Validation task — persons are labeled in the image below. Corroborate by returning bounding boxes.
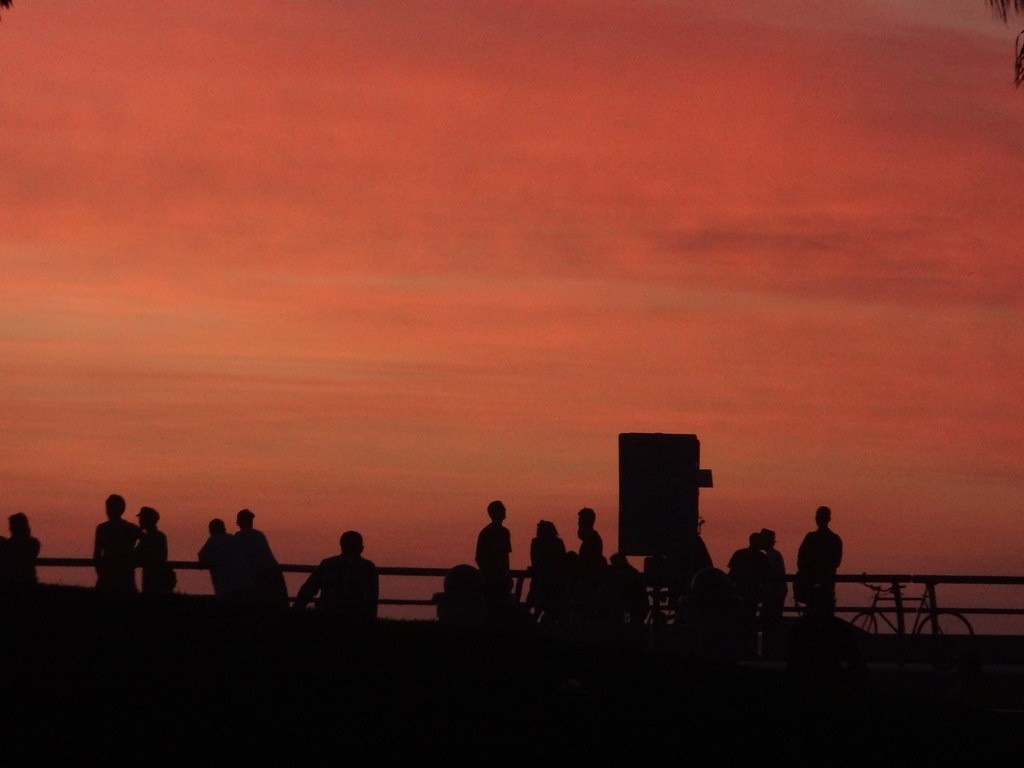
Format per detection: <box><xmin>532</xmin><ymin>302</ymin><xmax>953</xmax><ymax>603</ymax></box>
<box><xmin>523</xmin><ymin>520</ymin><xmax>565</xmax><ymax>622</ymax></box>
<box><xmin>92</xmin><ymin>494</ymin><xmax>139</xmax><ymax>591</ymax></box>
<box><xmin>3</xmin><ymin>513</ymin><xmax>41</xmax><ymax>587</ymax></box>
<box><xmin>131</xmin><ymin>506</ymin><xmax>179</xmax><ymax>594</ymax></box>
<box><xmin>793</xmin><ymin>504</ymin><xmax>842</xmax><ymax>618</ymax></box>
<box><xmin>233</xmin><ymin>509</ymin><xmax>288</xmax><ymax>602</ymax></box>
<box><xmin>756</xmin><ymin>528</ymin><xmax>788</xmax><ymax>617</ymax></box>
<box><xmin>475</xmin><ymin>501</ymin><xmax>514</xmax><ymax>620</ymax></box>
<box><xmin>567</xmin><ymin>507</ymin><xmax>653</xmax><ymax>628</ymax></box>
<box><xmin>725</xmin><ymin>532</ymin><xmax>766</xmax><ymax>622</ymax></box>
<box><xmin>198</xmin><ymin>515</ymin><xmax>250</xmax><ymax>593</ymax></box>
<box><xmin>292</xmin><ymin>526</ymin><xmax>380</xmax><ymax>618</ymax></box>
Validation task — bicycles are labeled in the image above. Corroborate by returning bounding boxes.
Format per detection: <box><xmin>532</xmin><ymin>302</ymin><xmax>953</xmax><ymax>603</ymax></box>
<box><xmin>848</xmin><ymin>572</ymin><xmax>978</xmax><ymax>663</ymax></box>
